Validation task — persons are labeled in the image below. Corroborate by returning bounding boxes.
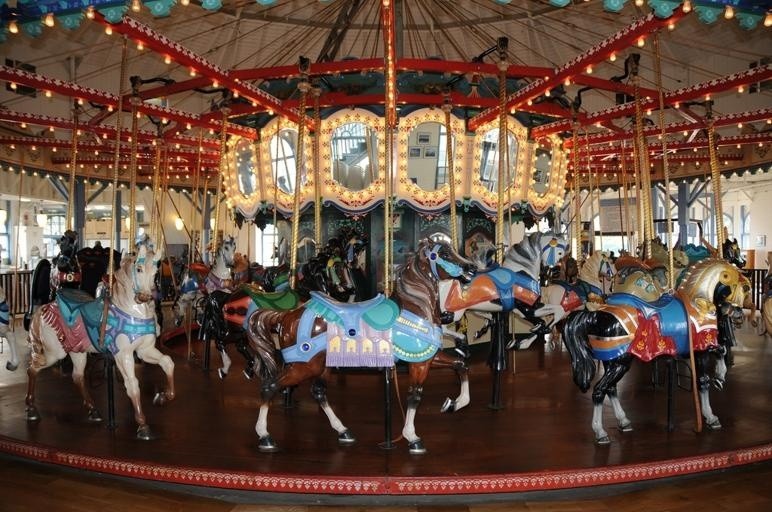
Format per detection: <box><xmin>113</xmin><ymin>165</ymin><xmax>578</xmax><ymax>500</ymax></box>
<box><xmin>278</xmin><ymin>176</ymin><xmax>287</xmax><ymax>190</ymax></box>
